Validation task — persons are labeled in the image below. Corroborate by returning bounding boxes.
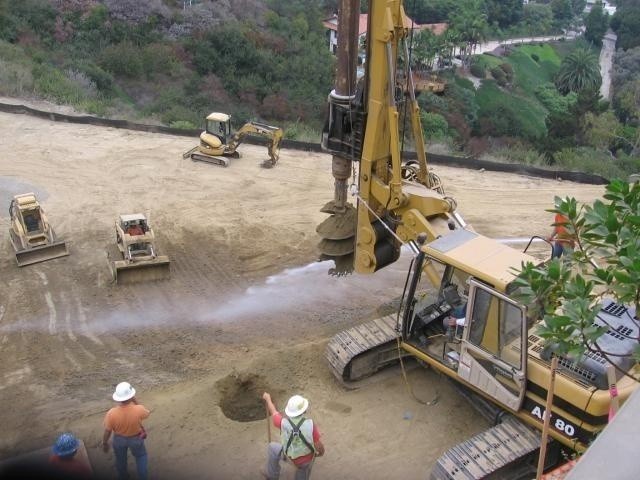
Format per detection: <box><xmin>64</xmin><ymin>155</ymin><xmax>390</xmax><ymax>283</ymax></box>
<box><xmin>102</xmin><ymin>381</ymin><xmax>151</xmax><ymax>480</ymax></box>
<box><xmin>441</xmin><ymin>274</ymin><xmax>488</xmax><ymax>336</ymax></box>
<box><xmin>218</xmin><ymin>122</ymin><xmax>225</xmax><ymax>133</ymax></box>
<box><xmin>546</xmin><ymin>204</ymin><xmax>580</xmax><ymax>267</ymax></box>
<box><xmin>47</xmin><ymin>430</ymin><xmax>93</xmax><ymax>480</ymax></box>
<box><xmin>125</xmin><ymin>222</ymin><xmax>146</xmax><ymax>251</ymax></box>
<box><xmin>260</xmin><ymin>390</ymin><xmax>325</xmax><ymax>480</ymax></box>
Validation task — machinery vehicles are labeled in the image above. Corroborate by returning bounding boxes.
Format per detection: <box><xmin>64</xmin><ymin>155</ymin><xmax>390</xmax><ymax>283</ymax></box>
<box><xmin>183</xmin><ymin>112</ymin><xmax>283</xmax><ymax>169</ymax></box>
<box><xmin>314</xmin><ymin>1</ymin><xmax>640</xmax><ymax>463</ymax></box>
<box><xmin>112</xmin><ymin>213</ymin><xmax>171</xmax><ymax>285</ymax></box>
<box><xmin>7</xmin><ymin>192</ymin><xmax>70</xmax><ymax>268</ymax></box>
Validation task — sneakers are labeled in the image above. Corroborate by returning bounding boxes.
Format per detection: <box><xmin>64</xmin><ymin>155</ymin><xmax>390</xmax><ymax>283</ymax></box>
<box><xmin>260</xmin><ymin>465</ymin><xmax>277</xmax><ymax>478</ymax></box>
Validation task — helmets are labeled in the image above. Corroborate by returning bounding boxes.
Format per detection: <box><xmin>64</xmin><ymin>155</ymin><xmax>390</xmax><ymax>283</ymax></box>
<box><xmin>285</xmin><ymin>395</ymin><xmax>308</xmax><ymax>418</ymax></box>
<box><xmin>52</xmin><ymin>433</ymin><xmax>80</xmax><ymax>457</ymax></box>
<box><xmin>112</xmin><ymin>381</ymin><xmax>136</xmax><ymax>402</ymax></box>
